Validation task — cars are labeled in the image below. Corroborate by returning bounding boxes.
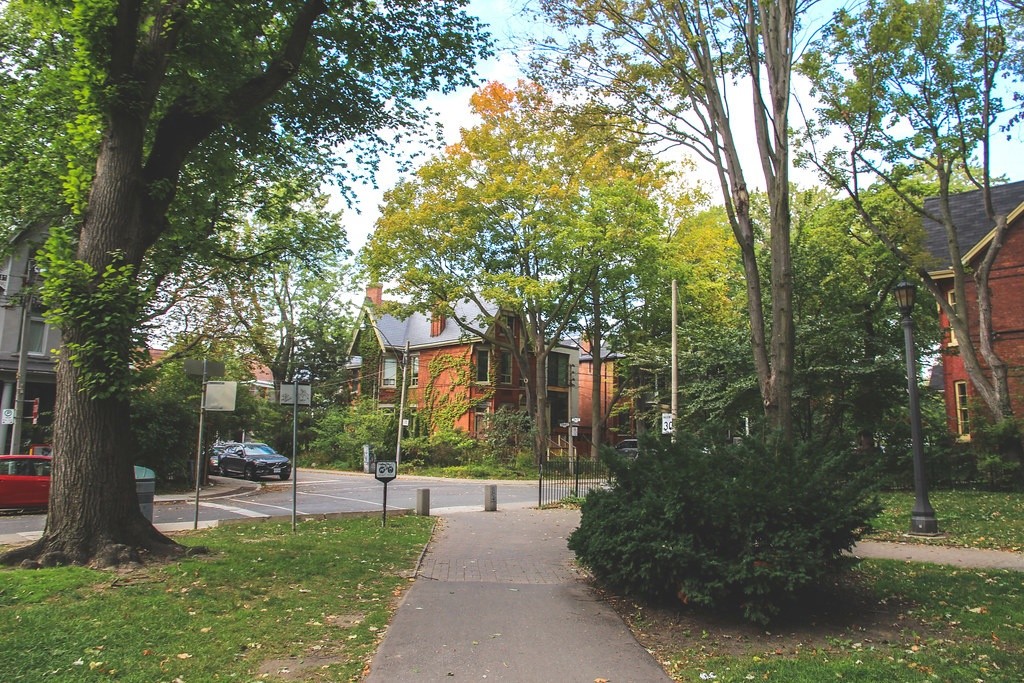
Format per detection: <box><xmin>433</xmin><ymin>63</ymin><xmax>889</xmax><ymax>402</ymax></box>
<box><xmin>614</xmin><ymin>438</ymin><xmax>657</xmax><ymax>460</ymax></box>
<box><xmin>0</xmin><ymin>454</ymin><xmax>52</xmax><ymax>506</ymax></box>
<box><xmin>208</xmin><ymin>443</ymin><xmax>227</xmax><ymax>475</ymax></box>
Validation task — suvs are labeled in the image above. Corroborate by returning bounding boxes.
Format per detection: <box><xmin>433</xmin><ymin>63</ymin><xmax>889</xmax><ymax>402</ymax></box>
<box><xmin>216</xmin><ymin>443</ymin><xmax>292</xmax><ymax>482</ymax></box>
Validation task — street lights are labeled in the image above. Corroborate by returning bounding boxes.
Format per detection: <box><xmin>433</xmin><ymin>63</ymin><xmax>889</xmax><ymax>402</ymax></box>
<box><xmin>890</xmin><ymin>279</ymin><xmax>943</xmax><ymax>537</ymax></box>
<box><xmin>358</xmin><ymin>320</ymin><xmax>411</xmax><ymax>472</ymax></box>
<box><xmin>192</xmin><ymin>338</ymin><xmax>213</xmax><ymax>530</ymax></box>
<box><xmin>6</xmin><ymin>260</ymin><xmax>50</xmax><ymax>473</ymax></box>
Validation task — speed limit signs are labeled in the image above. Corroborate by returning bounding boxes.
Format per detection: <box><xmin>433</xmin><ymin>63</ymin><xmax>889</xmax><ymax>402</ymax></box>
<box><xmin>661</xmin><ymin>413</ymin><xmax>673</xmax><ymax>435</ymax></box>
